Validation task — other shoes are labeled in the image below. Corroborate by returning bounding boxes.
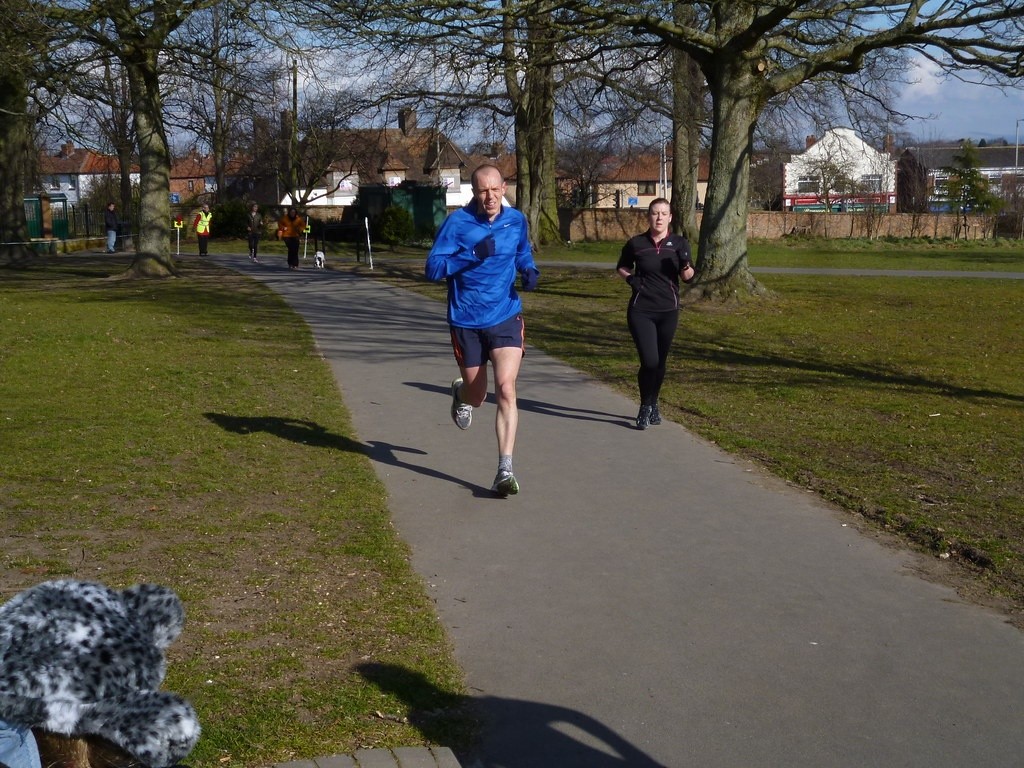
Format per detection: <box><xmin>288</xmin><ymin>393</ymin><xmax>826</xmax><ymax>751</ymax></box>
<box><xmin>253</xmin><ymin>257</ymin><xmax>258</xmax><ymax>263</ymax></box>
<box><xmin>289</xmin><ymin>265</ymin><xmax>297</xmax><ymax>270</ymax></box>
<box><xmin>107</xmin><ymin>249</ymin><xmax>118</xmax><ymax>254</ymax></box>
<box><xmin>249</xmin><ymin>252</ymin><xmax>252</xmax><ymax>260</ymax></box>
<box><xmin>200</xmin><ymin>252</ymin><xmax>209</xmax><ymax>256</ymax></box>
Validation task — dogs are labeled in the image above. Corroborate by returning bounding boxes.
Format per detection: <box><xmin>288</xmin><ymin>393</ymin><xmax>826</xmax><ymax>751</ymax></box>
<box><xmin>314</xmin><ymin>251</ymin><xmax>325</xmax><ymax>268</ymax></box>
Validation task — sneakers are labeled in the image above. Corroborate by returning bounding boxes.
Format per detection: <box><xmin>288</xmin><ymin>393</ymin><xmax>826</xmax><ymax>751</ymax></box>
<box><xmin>650</xmin><ymin>403</ymin><xmax>661</xmax><ymax>424</ymax></box>
<box><xmin>636</xmin><ymin>404</ymin><xmax>652</xmax><ymax>430</ymax></box>
<box><xmin>490</xmin><ymin>468</ymin><xmax>520</xmax><ymax>499</ymax></box>
<box><xmin>450</xmin><ymin>376</ymin><xmax>473</xmax><ymax>430</ymax></box>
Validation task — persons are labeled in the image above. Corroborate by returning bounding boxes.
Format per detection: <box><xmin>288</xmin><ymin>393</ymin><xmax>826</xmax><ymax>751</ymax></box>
<box><xmin>193</xmin><ymin>204</ymin><xmax>213</xmax><ymax>256</ymax></box>
<box><xmin>105</xmin><ymin>202</ymin><xmax>118</xmax><ymax>253</ymax></box>
<box><xmin>617</xmin><ymin>198</ymin><xmax>696</xmax><ymax>430</ymax></box>
<box><xmin>278</xmin><ymin>208</ymin><xmax>307</xmax><ymax>269</ymax></box>
<box><xmin>245</xmin><ymin>203</ymin><xmax>265</xmax><ymax>264</ymax></box>
<box><xmin>425</xmin><ymin>165</ymin><xmax>540</xmax><ymax>495</ymax></box>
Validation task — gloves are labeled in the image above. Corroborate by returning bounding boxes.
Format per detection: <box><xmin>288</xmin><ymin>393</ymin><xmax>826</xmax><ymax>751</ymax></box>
<box><xmin>676</xmin><ymin>249</ymin><xmax>689</xmax><ymax>269</ymax></box>
<box><xmin>625</xmin><ymin>275</ymin><xmax>643</xmax><ymax>293</ymax></box>
<box><xmin>520</xmin><ymin>270</ymin><xmax>537</xmax><ymax>291</ymax></box>
<box><xmin>473</xmin><ymin>232</ymin><xmax>496</xmax><ymax>260</ymax></box>
